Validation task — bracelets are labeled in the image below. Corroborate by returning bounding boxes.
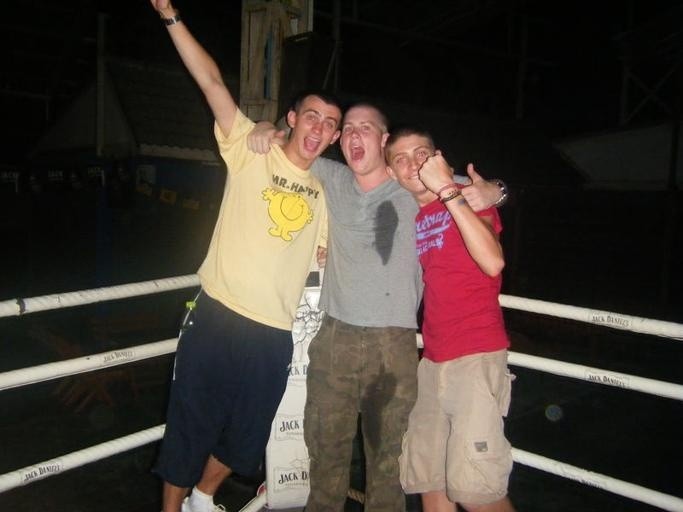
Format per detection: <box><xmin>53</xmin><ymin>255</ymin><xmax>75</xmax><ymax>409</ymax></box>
<box><xmin>161</xmin><ymin>8</ymin><xmax>180</xmax><ymax>25</ymax></box>
<box><xmin>437</xmin><ymin>184</ymin><xmax>461</xmax><ymax>204</ymax></box>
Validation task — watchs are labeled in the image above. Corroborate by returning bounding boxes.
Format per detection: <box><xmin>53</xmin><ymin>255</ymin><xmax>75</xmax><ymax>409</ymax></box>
<box><xmin>489</xmin><ymin>179</ymin><xmax>507</xmax><ymax>207</ymax></box>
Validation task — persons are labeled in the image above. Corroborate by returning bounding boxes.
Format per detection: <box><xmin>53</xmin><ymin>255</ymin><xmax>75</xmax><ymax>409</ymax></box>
<box><xmin>151</xmin><ymin>0</ymin><xmax>326</xmax><ymax>512</ymax></box>
<box><xmin>244</xmin><ymin>101</ymin><xmax>510</xmax><ymax>512</ymax></box>
<box><xmin>317</xmin><ymin>129</ymin><xmax>517</xmax><ymax>512</ymax></box>
